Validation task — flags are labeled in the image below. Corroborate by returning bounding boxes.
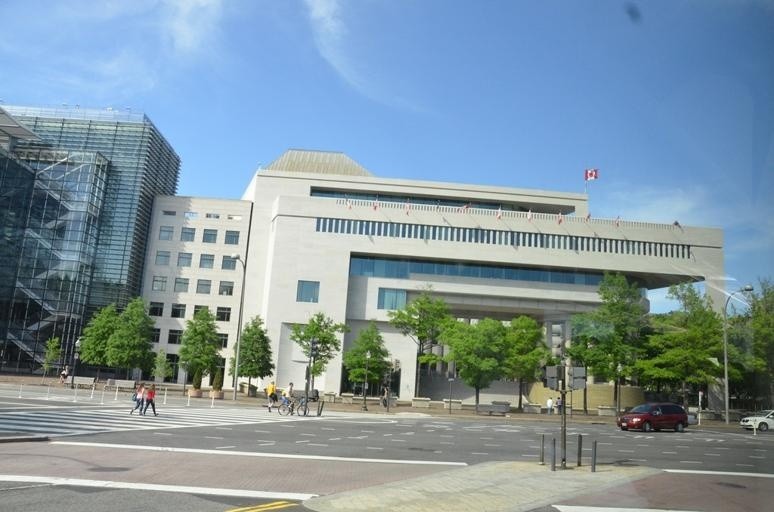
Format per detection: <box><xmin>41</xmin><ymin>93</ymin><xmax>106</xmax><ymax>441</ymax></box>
<box><xmin>585</xmin><ymin>169</ymin><xmax>598</xmax><ymax>181</ymax></box>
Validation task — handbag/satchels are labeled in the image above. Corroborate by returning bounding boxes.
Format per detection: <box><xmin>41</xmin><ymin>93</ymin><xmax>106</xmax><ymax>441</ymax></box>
<box><xmin>269</xmin><ymin>393</ymin><xmax>278</xmax><ymax>403</ymax></box>
<box><xmin>132</xmin><ymin>393</ymin><xmax>136</xmax><ymax>401</ymax></box>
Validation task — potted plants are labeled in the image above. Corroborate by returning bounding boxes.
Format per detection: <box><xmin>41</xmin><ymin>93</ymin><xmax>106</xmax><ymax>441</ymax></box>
<box><xmin>209</xmin><ymin>366</ymin><xmax>224</xmax><ymax>399</ymax></box>
<box><xmin>188</xmin><ymin>367</ymin><xmax>203</xmax><ymax>398</ymax></box>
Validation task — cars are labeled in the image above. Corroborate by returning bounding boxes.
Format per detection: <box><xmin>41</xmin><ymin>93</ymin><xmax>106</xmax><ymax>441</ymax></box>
<box><xmin>739</xmin><ymin>409</ymin><xmax>774</xmax><ymax>432</ymax></box>
<box><xmin>615</xmin><ymin>402</ymin><xmax>698</xmax><ymax>432</ymax></box>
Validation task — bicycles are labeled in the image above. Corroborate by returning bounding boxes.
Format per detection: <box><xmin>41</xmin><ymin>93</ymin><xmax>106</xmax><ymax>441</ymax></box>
<box><xmin>277</xmin><ymin>395</ymin><xmax>309</xmax><ymax>417</ymax></box>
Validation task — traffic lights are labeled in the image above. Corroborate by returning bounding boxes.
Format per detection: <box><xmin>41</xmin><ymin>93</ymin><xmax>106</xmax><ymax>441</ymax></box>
<box><xmin>545</xmin><ymin>320</ymin><xmax>586</xmax><ymax>392</ymax></box>
<box><xmin>309</xmin><ymin>338</ymin><xmax>320</xmax><ymax>357</ymax></box>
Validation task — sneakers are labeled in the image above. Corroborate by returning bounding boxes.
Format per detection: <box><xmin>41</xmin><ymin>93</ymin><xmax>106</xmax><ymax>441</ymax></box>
<box><xmin>130</xmin><ymin>412</ymin><xmax>159</xmax><ymax>416</ymax></box>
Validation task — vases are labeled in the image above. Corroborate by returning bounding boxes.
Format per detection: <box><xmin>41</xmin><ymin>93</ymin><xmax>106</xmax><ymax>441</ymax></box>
<box><xmin>696</xmin><ymin>409</ymin><xmax>742</xmax><ymax>421</ymax></box>
<box><xmin>239</xmin><ymin>381</ymin><xmax>618</xmax><ymax>418</ymax></box>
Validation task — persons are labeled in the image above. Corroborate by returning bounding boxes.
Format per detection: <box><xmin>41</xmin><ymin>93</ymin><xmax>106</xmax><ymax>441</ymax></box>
<box><xmin>143</xmin><ymin>384</ymin><xmax>159</xmax><ymax>416</ymax></box>
<box><xmin>267</xmin><ymin>380</ymin><xmax>276</xmax><ymax>412</ymax></box>
<box><xmin>381</xmin><ymin>384</ymin><xmax>389</xmax><ymax>407</ymax></box>
<box><xmin>383</xmin><ymin>385</ymin><xmax>388</xmax><ymax>409</ymax></box>
<box><xmin>546</xmin><ymin>397</ymin><xmax>554</xmax><ymax>414</ymax></box>
<box><xmin>285</xmin><ymin>382</ymin><xmax>295</xmax><ymax>415</ymax></box>
<box><xmin>556</xmin><ymin>397</ymin><xmax>562</xmax><ymax>415</ymax></box>
<box><xmin>130</xmin><ymin>382</ymin><xmax>145</xmax><ymax>416</ymax></box>
<box><xmin>61</xmin><ymin>369</ymin><xmax>67</xmax><ymax>384</ymax></box>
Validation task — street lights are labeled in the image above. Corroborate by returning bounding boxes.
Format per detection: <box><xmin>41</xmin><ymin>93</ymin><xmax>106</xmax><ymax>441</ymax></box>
<box><xmin>361</xmin><ymin>350</ymin><xmax>372</xmax><ymax>412</ymax></box>
<box><xmin>723</xmin><ymin>285</ymin><xmax>754</xmax><ymax>424</ymax></box>
<box><xmin>70</xmin><ymin>338</ymin><xmax>81</xmax><ymax>388</ymax></box>
<box><xmin>232</xmin><ymin>253</ymin><xmax>246</xmax><ymax>401</ymax></box>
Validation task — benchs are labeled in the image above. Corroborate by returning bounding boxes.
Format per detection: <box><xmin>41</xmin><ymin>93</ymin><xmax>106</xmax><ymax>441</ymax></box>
<box><xmin>64</xmin><ymin>375</ymin><xmax>96</xmax><ymax>390</ymax></box>
<box><xmin>104</xmin><ymin>378</ymin><xmax>136</xmax><ymax>393</ymax></box>
